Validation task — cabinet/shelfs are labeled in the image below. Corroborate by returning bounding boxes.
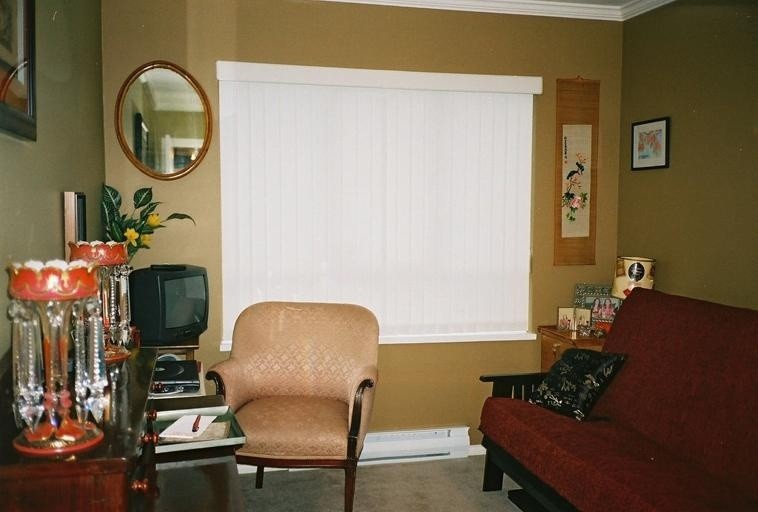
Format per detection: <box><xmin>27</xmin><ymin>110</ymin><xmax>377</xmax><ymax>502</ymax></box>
<box><xmin>536</xmin><ymin>326</ymin><xmax>608</xmax><ymax>372</ymax></box>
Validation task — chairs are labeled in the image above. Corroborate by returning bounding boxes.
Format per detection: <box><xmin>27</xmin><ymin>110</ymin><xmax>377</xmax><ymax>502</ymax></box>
<box><xmin>205</xmin><ymin>296</ymin><xmax>381</xmax><ymax>512</ymax></box>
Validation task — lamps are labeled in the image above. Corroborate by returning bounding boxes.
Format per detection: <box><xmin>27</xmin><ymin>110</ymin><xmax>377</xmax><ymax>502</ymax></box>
<box><xmin>611</xmin><ymin>255</ymin><xmax>656</xmax><ymax>302</ymax></box>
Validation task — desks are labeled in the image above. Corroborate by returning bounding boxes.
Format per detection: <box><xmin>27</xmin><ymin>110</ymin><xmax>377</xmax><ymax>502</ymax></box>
<box><xmin>0</xmin><ymin>330</ymin><xmax>244</xmax><ymax>512</ymax></box>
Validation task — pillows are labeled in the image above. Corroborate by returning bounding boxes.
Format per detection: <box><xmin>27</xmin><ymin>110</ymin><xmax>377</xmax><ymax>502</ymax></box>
<box><xmin>532</xmin><ymin>345</ymin><xmax>629</xmax><ymax>416</ymax></box>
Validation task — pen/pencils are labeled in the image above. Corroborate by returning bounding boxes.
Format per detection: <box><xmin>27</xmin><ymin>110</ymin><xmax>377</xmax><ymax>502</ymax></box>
<box><xmin>193</xmin><ymin>415</ymin><xmax>201</xmax><ymax>432</ymax></box>
<box><xmin>151</xmin><ymin>265</ymin><xmax>186</xmax><ymax>271</ymax></box>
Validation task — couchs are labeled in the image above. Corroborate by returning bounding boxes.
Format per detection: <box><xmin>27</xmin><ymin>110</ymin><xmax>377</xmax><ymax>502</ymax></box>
<box><xmin>477</xmin><ymin>286</ymin><xmax>757</xmax><ymax>512</ymax></box>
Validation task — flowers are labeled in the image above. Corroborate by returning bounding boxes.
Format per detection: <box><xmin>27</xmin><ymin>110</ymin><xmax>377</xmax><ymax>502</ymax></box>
<box><xmin>100</xmin><ymin>183</ymin><xmax>197</xmax><ymax>265</ymax></box>
<box><xmin>562</xmin><ymin>152</ymin><xmax>588</xmax><ymax>222</ymax></box>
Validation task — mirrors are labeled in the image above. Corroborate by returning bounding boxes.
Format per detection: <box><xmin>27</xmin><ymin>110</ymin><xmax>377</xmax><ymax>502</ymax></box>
<box><xmin>114</xmin><ymin>59</ymin><xmax>213</xmax><ymax>181</ymax></box>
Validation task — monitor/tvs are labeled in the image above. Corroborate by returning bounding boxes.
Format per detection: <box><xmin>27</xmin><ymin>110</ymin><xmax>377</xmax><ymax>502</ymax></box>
<box><xmin>127</xmin><ymin>265</ymin><xmax>208</xmax><ymax>346</ymax></box>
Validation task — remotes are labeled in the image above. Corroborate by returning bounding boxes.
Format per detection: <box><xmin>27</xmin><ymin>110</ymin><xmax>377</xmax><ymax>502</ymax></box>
<box><xmin>151</xmin><ymin>263</ymin><xmax>186</xmax><ymax>271</ymax></box>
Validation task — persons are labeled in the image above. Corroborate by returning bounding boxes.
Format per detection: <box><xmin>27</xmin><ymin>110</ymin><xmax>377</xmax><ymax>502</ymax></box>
<box><xmin>587</xmin><ymin>297</ymin><xmax>602</xmax><ymax>319</ymax></box>
<box><xmin>575</xmin><ymin>315</ymin><xmax>587</xmax><ymax>327</ymax></box>
<box><xmin>558</xmin><ymin>314</ymin><xmax>569</xmax><ymax>328</ymax></box>
<box><xmin>600</xmin><ymin>298</ymin><xmax>615</xmax><ymax>320</ymax></box>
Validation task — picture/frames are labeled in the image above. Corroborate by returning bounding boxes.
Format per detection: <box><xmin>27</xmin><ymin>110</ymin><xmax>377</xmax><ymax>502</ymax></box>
<box><xmin>135</xmin><ymin>113</ymin><xmax>151</xmax><ymax>171</ymax></box>
<box><xmin>575</xmin><ymin>281</ymin><xmax>620</xmax><ymax>331</ymax></box>
<box><xmin>630</xmin><ymin>116</ymin><xmax>672</xmax><ymax>171</ymax></box>
<box><xmin>0</xmin><ymin>0</ymin><xmax>39</xmax><ymax>140</ymax></box>
